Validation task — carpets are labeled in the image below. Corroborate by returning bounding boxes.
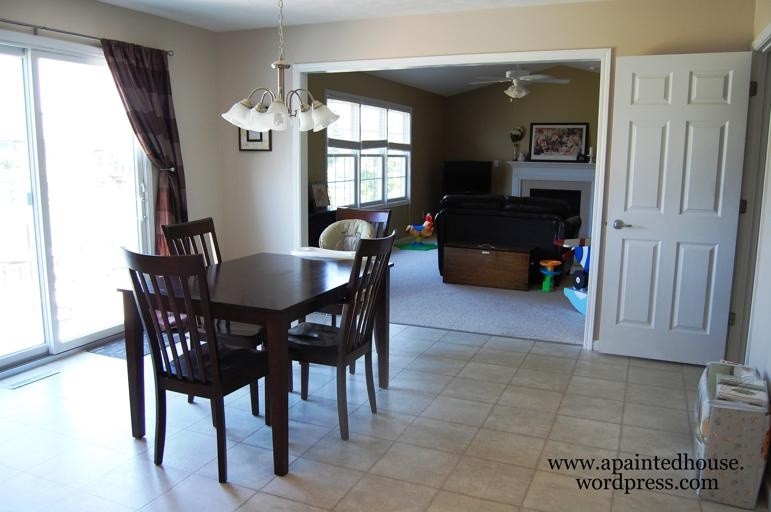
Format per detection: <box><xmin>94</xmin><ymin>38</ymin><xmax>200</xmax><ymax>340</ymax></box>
<box><xmin>386</xmin><ymin>229</ymin><xmax>584</xmax><ymax>348</ymax></box>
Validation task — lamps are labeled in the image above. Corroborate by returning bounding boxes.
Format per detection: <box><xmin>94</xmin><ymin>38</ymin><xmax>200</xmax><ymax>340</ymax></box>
<box><xmin>504</xmin><ymin>81</ymin><xmax>531</xmax><ymax>101</ymax></box>
<box><xmin>218</xmin><ymin>0</ymin><xmax>338</xmax><ymax>135</ymax></box>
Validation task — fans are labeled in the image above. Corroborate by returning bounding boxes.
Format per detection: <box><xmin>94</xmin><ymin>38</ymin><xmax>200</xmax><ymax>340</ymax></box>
<box><xmin>466</xmin><ymin>62</ymin><xmax>571</xmax><ymax>85</ymax></box>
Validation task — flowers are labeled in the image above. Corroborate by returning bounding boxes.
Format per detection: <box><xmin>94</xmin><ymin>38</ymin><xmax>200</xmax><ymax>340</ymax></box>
<box><xmin>506</xmin><ymin>126</ymin><xmax>525</xmax><ymax>141</ymax></box>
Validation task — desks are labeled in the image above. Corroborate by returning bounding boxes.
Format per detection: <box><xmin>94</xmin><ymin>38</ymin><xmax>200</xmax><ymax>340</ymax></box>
<box><xmin>115</xmin><ymin>251</ymin><xmax>395</xmax><ymax>478</ymax></box>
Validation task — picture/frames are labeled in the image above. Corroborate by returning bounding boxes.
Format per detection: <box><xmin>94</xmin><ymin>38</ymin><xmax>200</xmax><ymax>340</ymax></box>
<box><xmin>237</xmin><ymin>105</ymin><xmax>271</xmax><ymax>153</ymax></box>
<box><xmin>528</xmin><ymin>121</ymin><xmax>590</xmax><ymax>163</ymax></box>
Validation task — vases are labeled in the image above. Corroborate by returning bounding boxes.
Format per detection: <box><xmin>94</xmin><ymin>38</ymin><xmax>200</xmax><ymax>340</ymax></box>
<box><xmin>512</xmin><ymin>142</ymin><xmax>518</xmax><ymax>161</ymax></box>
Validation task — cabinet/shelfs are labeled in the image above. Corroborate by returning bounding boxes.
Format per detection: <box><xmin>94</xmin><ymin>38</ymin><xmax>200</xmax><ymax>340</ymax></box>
<box><xmin>119</xmin><ymin>244</ymin><xmax>272</xmax><ymax>485</ymax></box>
<box><xmin>285</xmin><ymin>230</ymin><xmax>405</xmax><ymax>446</ymax></box>
<box><xmin>159</xmin><ymin>216</ymin><xmax>292</xmax><ymax>418</ymax></box>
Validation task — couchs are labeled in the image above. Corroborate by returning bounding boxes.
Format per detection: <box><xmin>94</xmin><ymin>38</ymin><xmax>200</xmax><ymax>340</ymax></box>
<box><xmin>433</xmin><ymin>192</ymin><xmax>582</xmax><ymax>288</ymax></box>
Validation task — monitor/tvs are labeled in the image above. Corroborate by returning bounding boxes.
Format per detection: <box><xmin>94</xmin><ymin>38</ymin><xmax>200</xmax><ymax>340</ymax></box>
<box><xmin>309</xmin><ymin>181</ymin><xmax>330</xmax><ymax>209</ymax></box>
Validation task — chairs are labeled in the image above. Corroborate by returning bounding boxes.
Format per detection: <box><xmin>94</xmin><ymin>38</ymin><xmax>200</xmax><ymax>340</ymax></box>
<box><xmin>296</xmin><ymin>206</ymin><xmax>394</xmax><ymax>375</ymax></box>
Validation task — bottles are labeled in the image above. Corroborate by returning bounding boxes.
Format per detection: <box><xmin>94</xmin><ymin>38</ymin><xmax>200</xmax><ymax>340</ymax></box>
<box><xmin>518</xmin><ymin>153</ymin><xmax>526</xmax><ymax>162</ymax></box>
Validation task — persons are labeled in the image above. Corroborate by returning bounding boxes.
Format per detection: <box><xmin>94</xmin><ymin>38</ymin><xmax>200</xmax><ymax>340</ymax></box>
<box><xmin>553</xmin><ymin>238</ymin><xmax>590</xmax><ymax>315</ymax></box>
